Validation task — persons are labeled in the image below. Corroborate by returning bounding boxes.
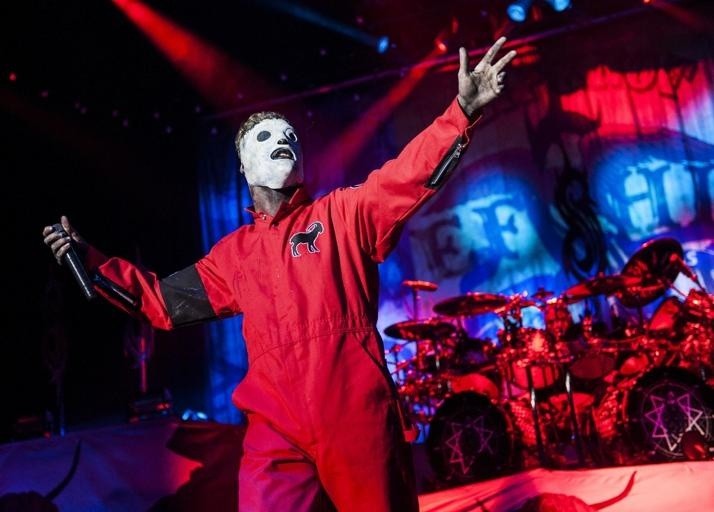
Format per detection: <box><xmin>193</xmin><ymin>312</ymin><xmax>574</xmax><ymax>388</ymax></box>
<box><xmin>40</xmin><ymin>32</ymin><xmax>518</xmax><ymax>512</ymax></box>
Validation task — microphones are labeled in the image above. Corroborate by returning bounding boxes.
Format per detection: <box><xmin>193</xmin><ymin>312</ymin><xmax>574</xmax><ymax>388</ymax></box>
<box><xmin>402</xmin><ymin>278</ymin><xmax>437</xmax><ymax>293</ymax></box>
<box><xmin>390</xmin><ymin>355</ymin><xmax>417</xmax><ymax>375</ymax></box>
<box><xmin>670</xmin><ymin>253</ymin><xmax>698</xmax><ymax>282</ymax></box>
<box><xmin>49</xmin><ymin>217</ymin><xmax>95</xmax><ymax>302</ymax></box>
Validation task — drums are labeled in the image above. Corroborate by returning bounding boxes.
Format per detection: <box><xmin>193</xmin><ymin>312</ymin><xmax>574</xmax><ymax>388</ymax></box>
<box><xmin>500</xmin><ymin>331</ymin><xmax>564</xmax><ymax>390</ymax></box>
<box><xmin>672</xmin><ymin>289</ymin><xmax>714</xmax><ymax>329</ymax></box>
<box><xmin>568</xmin><ymin>335</ymin><xmax>618</xmax><ymax>379</ymax></box>
<box><xmin>447</xmin><ymin>339</ymin><xmax>501</xmax><ymax>400</ymax></box>
<box><xmin>591</xmin><ymin>365</ymin><xmax>713</xmax><ymax>464</ymax></box>
<box><xmin>426</xmin><ymin>392</ymin><xmax>541</xmax><ymax>486</ymax></box>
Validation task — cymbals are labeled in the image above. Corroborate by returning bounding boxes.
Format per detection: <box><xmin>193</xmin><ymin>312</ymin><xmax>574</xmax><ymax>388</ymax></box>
<box><xmin>384</xmin><ymin>318</ymin><xmax>453</xmax><ymax>340</ymax></box>
<box><xmin>433</xmin><ymin>293</ymin><xmax>510</xmax><ymax>315</ymax></box>
<box><xmin>568</xmin><ymin>274</ymin><xmax>620</xmax><ymax>297</ymax></box>
<box><xmin>403</xmin><ymin>279</ymin><xmax>437</xmax><ymax>291</ymax></box>
<box><xmin>616</xmin><ymin>237</ymin><xmax>685</xmax><ymax>309</ymax></box>
